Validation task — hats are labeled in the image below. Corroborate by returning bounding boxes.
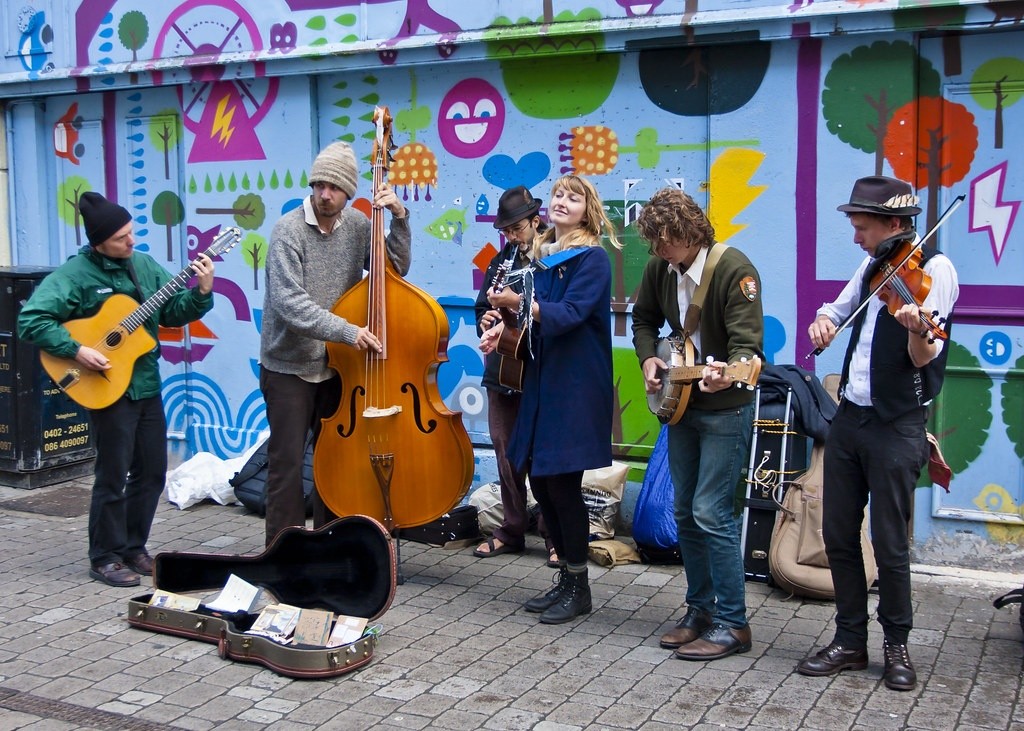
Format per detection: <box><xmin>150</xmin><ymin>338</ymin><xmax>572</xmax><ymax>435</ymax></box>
<box><xmin>308</xmin><ymin>141</ymin><xmax>357</xmax><ymax>199</ymax></box>
<box><xmin>493</xmin><ymin>186</ymin><xmax>542</xmax><ymax>229</ymax></box>
<box><xmin>79</xmin><ymin>192</ymin><xmax>132</xmax><ymax>247</ymax></box>
<box><xmin>837</xmin><ymin>175</ymin><xmax>922</xmax><ymax>216</ymax></box>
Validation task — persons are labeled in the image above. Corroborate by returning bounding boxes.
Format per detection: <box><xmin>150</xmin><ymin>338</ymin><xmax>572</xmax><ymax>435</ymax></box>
<box><xmin>259</xmin><ymin>141</ymin><xmax>410</xmax><ymax>550</ymax></box>
<box><xmin>796</xmin><ymin>176</ymin><xmax>959</xmax><ymax>692</ymax></box>
<box><xmin>630</xmin><ymin>186</ymin><xmax>764</xmax><ymax>661</ymax></box>
<box><xmin>478</xmin><ymin>171</ymin><xmax>628</xmax><ymax>624</ymax></box>
<box><xmin>17</xmin><ymin>192</ymin><xmax>214</xmax><ymax>588</ymax></box>
<box><xmin>472</xmin><ymin>185</ymin><xmax>561</xmax><ymax>569</ymax></box>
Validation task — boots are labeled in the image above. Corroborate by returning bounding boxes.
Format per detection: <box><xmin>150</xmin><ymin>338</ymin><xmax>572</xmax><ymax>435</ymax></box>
<box><xmin>540</xmin><ymin>571</ymin><xmax>592</xmax><ymax>624</ymax></box>
<box><xmin>523</xmin><ymin>565</ymin><xmax>570</xmax><ymax>613</ymax></box>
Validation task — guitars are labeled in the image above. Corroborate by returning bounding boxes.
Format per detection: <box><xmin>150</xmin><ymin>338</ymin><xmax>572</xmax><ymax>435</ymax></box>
<box><xmin>37</xmin><ymin>225</ymin><xmax>242</xmax><ymax>410</ymax></box>
<box><xmin>645</xmin><ymin>329</ymin><xmax>763</xmax><ymax>427</ymax></box>
<box><xmin>491</xmin><ymin>258</ymin><xmax>530</xmax><ymax>393</ymax></box>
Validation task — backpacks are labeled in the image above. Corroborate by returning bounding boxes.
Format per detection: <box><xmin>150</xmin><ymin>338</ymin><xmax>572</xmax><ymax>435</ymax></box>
<box><xmin>234</xmin><ymin>426</ymin><xmax>315</xmax><ymax>515</ymax></box>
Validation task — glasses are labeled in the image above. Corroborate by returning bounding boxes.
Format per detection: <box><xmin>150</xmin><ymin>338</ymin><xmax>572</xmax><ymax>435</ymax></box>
<box><xmin>498</xmin><ymin>222</ymin><xmax>530</xmax><ymax>238</ymax></box>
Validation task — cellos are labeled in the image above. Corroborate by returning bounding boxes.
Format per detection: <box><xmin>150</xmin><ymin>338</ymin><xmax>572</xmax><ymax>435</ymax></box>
<box><xmin>311</xmin><ymin>103</ymin><xmax>477</xmax><ymax>587</ymax></box>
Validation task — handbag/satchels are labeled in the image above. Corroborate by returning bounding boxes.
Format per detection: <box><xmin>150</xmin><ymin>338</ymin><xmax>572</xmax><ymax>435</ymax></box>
<box><xmin>580</xmin><ymin>461</ymin><xmax>631</xmax><ymax>539</ymax></box>
<box><xmin>468</xmin><ymin>471</ymin><xmax>539</xmax><ymax>533</ymax></box>
<box><xmin>587</xmin><ymin>539</ymin><xmax>640</xmax><ymax>570</ymax></box>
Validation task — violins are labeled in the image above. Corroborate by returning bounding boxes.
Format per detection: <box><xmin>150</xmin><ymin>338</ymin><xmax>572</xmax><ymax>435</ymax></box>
<box><xmin>869</xmin><ymin>238</ymin><xmax>948</xmax><ymax>345</ymax></box>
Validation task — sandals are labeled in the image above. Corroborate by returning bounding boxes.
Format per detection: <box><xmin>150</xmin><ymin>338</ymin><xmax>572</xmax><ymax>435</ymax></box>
<box><xmin>544</xmin><ymin>540</ymin><xmax>559</xmax><ymax>568</ymax></box>
<box><xmin>473</xmin><ymin>538</ymin><xmax>525</xmax><ymax>557</ymax></box>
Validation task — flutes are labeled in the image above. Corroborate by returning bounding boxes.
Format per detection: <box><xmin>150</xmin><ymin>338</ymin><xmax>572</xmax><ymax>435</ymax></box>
<box><xmin>490</xmin><ymin>244</ymin><xmax>520</xmax><ymax>329</ymax></box>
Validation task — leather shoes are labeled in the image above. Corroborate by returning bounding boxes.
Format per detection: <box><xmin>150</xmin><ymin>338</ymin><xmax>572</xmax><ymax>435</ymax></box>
<box><xmin>88</xmin><ymin>561</ymin><xmax>140</xmax><ymax>586</ymax></box>
<box><xmin>659</xmin><ymin>610</ymin><xmax>712</xmax><ymax>647</ymax></box>
<box><xmin>677</xmin><ymin>623</ymin><xmax>751</xmax><ymax>661</ymax></box>
<box><xmin>882</xmin><ymin>638</ymin><xmax>918</xmax><ymax>690</ymax></box>
<box><xmin>127</xmin><ymin>552</ymin><xmax>154</xmax><ymax>576</ymax></box>
<box><xmin>798</xmin><ymin>642</ymin><xmax>868</xmax><ymax>676</ymax></box>
